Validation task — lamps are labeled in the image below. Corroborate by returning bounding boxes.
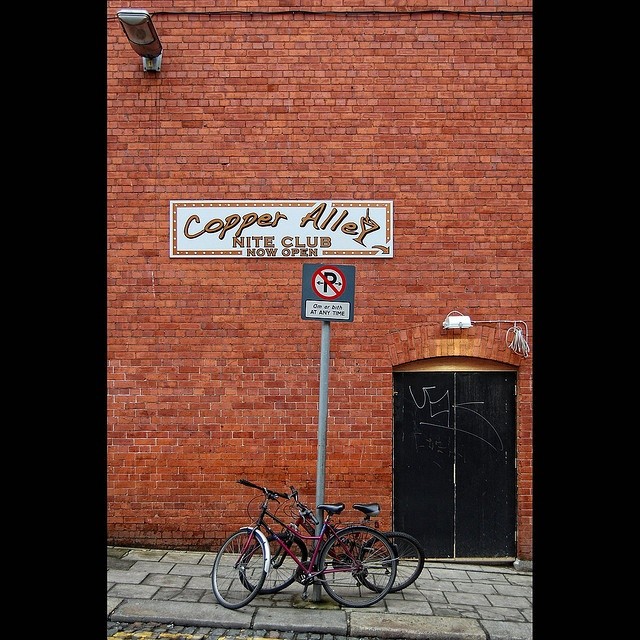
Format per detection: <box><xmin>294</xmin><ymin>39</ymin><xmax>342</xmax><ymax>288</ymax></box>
<box><xmin>116</xmin><ymin>9</ymin><xmax>163</xmax><ymax>72</ymax></box>
<box><xmin>443</xmin><ymin>315</ymin><xmax>471</xmax><ymax>329</ymax></box>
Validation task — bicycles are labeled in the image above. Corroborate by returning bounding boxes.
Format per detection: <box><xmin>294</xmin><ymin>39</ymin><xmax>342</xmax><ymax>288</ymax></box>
<box><xmin>241</xmin><ymin>484</ymin><xmax>425</xmax><ymax>595</ymax></box>
<box><xmin>212</xmin><ymin>475</ymin><xmax>398</xmax><ymax>611</ymax></box>
